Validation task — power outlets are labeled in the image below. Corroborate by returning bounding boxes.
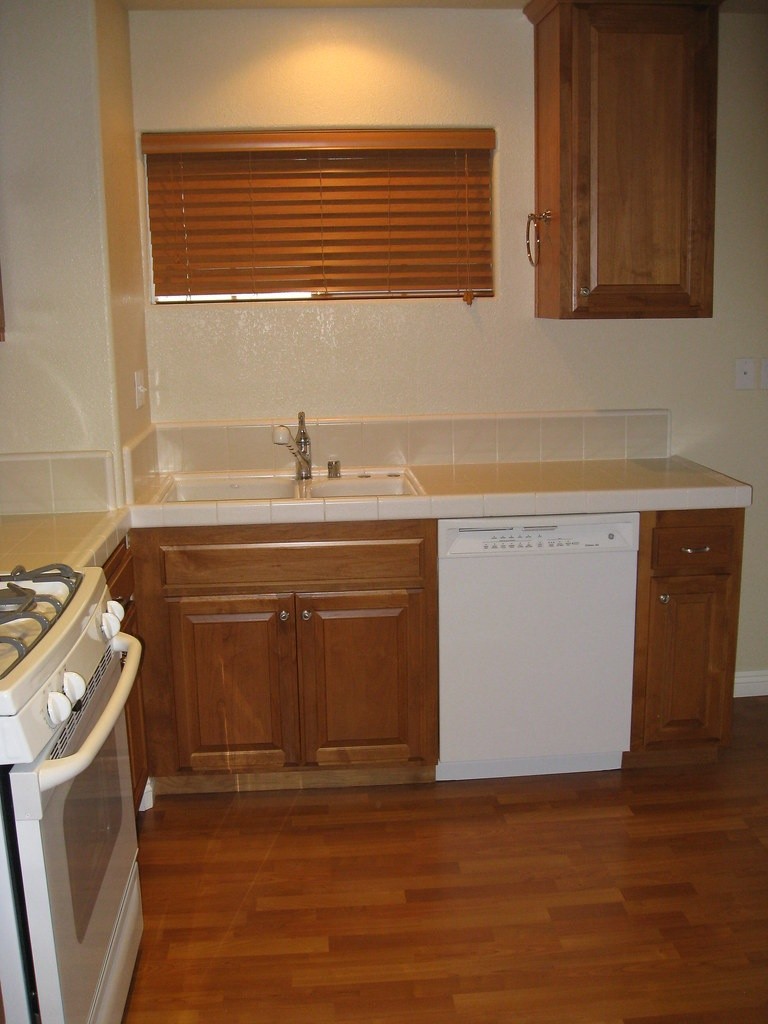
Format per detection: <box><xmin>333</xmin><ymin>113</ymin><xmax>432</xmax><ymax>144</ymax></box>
<box><xmin>135</xmin><ymin>369</ymin><xmax>148</xmax><ymax>409</ymax></box>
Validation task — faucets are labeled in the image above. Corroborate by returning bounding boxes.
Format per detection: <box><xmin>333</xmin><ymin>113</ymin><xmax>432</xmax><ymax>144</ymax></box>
<box><xmin>271</xmin><ymin>410</ymin><xmax>312</xmax><ymax>480</ymax></box>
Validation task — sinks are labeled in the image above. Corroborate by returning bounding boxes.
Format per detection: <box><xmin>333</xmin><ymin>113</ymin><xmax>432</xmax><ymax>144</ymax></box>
<box><xmin>150</xmin><ymin>472</ymin><xmax>305</xmax><ymax>504</ymax></box>
<box><xmin>303</xmin><ymin>463</ymin><xmax>430</xmax><ymax>503</ymax></box>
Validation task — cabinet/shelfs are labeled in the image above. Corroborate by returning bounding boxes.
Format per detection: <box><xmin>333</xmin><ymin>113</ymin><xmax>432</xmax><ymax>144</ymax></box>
<box><xmin>521</xmin><ymin>0</ymin><xmax>725</xmax><ymax>320</ymax></box>
<box><xmin>622</xmin><ymin>507</ymin><xmax>745</xmax><ymax>769</ymax></box>
<box><xmin>129</xmin><ymin>519</ymin><xmax>440</xmax><ymax>796</ymax></box>
